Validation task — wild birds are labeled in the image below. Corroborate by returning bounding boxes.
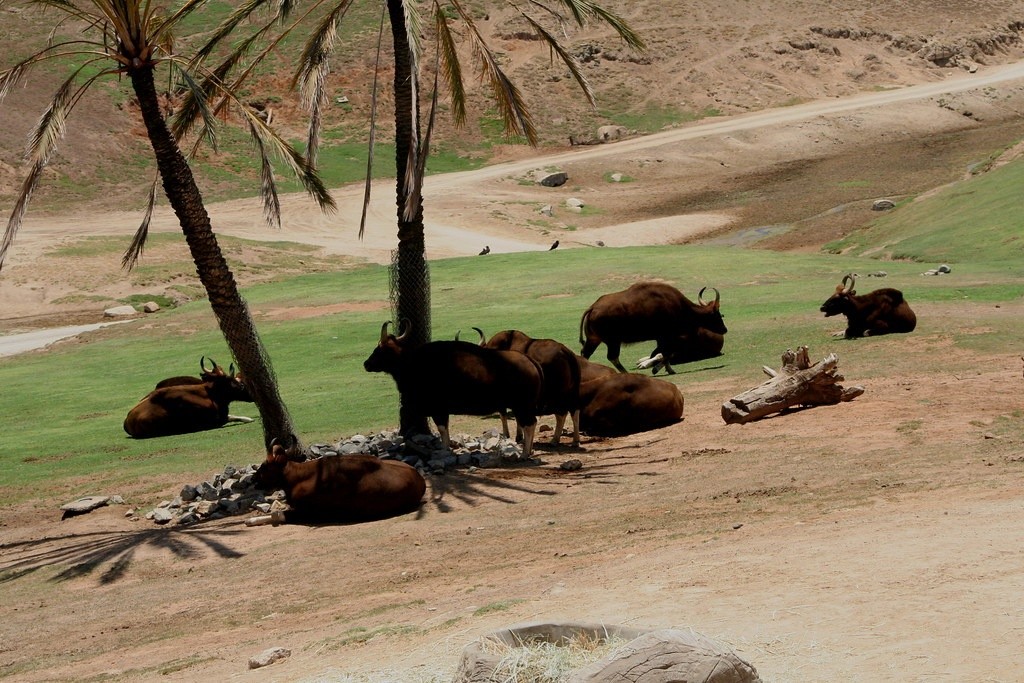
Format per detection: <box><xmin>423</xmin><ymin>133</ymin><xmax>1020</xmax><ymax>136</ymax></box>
<box><xmin>479</xmin><ymin>246</ymin><xmax>490</xmax><ymax>255</ymax></box>
<box><xmin>549</xmin><ymin>240</ymin><xmax>560</xmax><ymax>252</ymax></box>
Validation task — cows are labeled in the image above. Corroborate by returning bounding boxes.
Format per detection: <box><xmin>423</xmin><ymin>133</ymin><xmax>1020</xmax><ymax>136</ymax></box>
<box><xmin>244</xmin><ymin>433</ymin><xmax>426</xmax><ymax>526</ymax></box>
<box><xmin>362</xmin><ymin>317</ymin><xmax>684</xmax><ymax>466</ymax></box>
<box><xmin>819</xmin><ymin>273</ymin><xmax>917</xmax><ymax>341</ymax></box>
<box><xmin>123</xmin><ymin>355</ymin><xmax>255</xmax><ymax>439</ymax></box>
<box><xmin>578</xmin><ymin>281</ymin><xmax>727</xmax><ymax>376</ymax></box>
